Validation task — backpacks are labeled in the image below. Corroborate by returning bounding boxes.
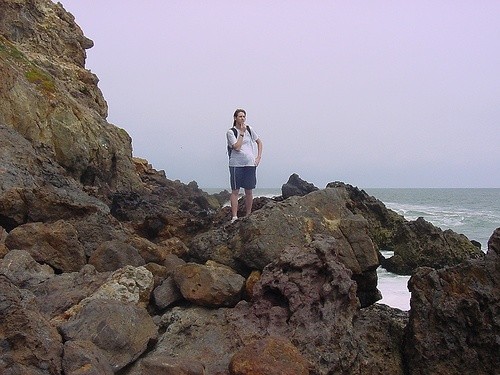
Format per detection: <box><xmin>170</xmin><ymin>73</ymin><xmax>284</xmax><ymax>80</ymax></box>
<box><xmin>226</xmin><ymin>125</ymin><xmax>251</xmax><ymax>157</ymax></box>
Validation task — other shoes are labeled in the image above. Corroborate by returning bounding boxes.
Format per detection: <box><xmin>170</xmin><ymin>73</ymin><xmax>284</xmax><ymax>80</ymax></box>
<box><xmin>231</xmin><ymin>216</ymin><xmax>237</xmax><ymax>223</ymax></box>
